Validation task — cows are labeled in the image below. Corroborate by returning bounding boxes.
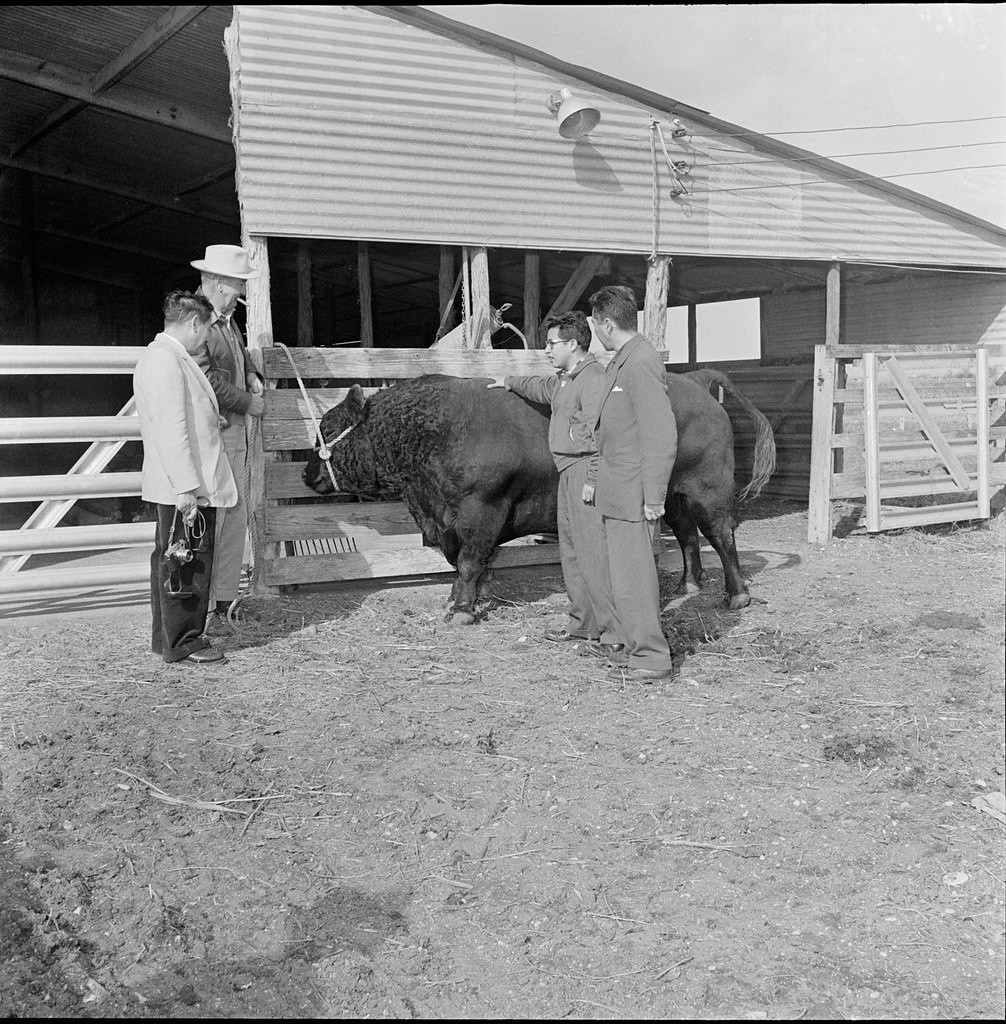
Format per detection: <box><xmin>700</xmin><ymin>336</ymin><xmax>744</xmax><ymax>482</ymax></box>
<box><xmin>301</xmin><ymin>368</ymin><xmax>777</xmax><ymax>625</ymax></box>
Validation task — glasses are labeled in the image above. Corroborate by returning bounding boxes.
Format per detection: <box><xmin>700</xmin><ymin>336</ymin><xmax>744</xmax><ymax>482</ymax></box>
<box><xmin>545</xmin><ymin>340</ymin><xmax>569</xmax><ymax>348</ymax></box>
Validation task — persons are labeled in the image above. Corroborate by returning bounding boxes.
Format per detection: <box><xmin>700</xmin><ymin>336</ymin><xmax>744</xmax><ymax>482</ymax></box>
<box><xmin>487</xmin><ymin>308</ymin><xmax>626</xmax><ymax>657</ymax></box>
<box><xmin>134</xmin><ymin>289</ymin><xmax>230</xmax><ymax>662</ymax></box>
<box><xmin>579</xmin><ymin>285</ymin><xmax>679</xmax><ymax>680</ymax></box>
<box><xmin>190</xmin><ymin>244</ymin><xmax>270</xmax><ymax>620</ymax></box>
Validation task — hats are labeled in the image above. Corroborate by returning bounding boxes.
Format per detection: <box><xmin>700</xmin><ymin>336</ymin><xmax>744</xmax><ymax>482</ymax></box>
<box><xmin>190</xmin><ymin>244</ymin><xmax>261</xmax><ymax>279</ymax></box>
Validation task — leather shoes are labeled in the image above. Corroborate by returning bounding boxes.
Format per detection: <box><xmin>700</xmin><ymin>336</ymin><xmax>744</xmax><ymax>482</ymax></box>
<box><xmin>174</xmin><ymin>647</ymin><xmax>228</xmax><ymax>668</ymax></box>
<box><xmin>608</xmin><ymin>668</ymin><xmax>673</xmax><ymax>686</ymax></box>
<box><xmin>545</xmin><ymin>630</ymin><xmax>599</xmax><ymax>643</ymax></box>
<box><xmin>214</xmin><ymin>603</ymin><xmax>261</xmax><ymax>623</ymax></box>
<box><xmin>586</xmin><ymin>642</ymin><xmax>626</xmax><ymax>658</ymax></box>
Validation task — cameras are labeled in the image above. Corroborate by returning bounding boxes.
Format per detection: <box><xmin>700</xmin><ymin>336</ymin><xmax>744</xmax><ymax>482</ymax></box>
<box><xmin>165</xmin><ymin>539</ymin><xmax>194</xmax><ymax>573</ymax></box>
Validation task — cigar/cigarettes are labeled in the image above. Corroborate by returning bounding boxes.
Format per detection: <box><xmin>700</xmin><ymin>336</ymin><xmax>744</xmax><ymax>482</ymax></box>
<box><xmin>236</xmin><ymin>297</ymin><xmax>250</xmax><ymax>308</ymax></box>
